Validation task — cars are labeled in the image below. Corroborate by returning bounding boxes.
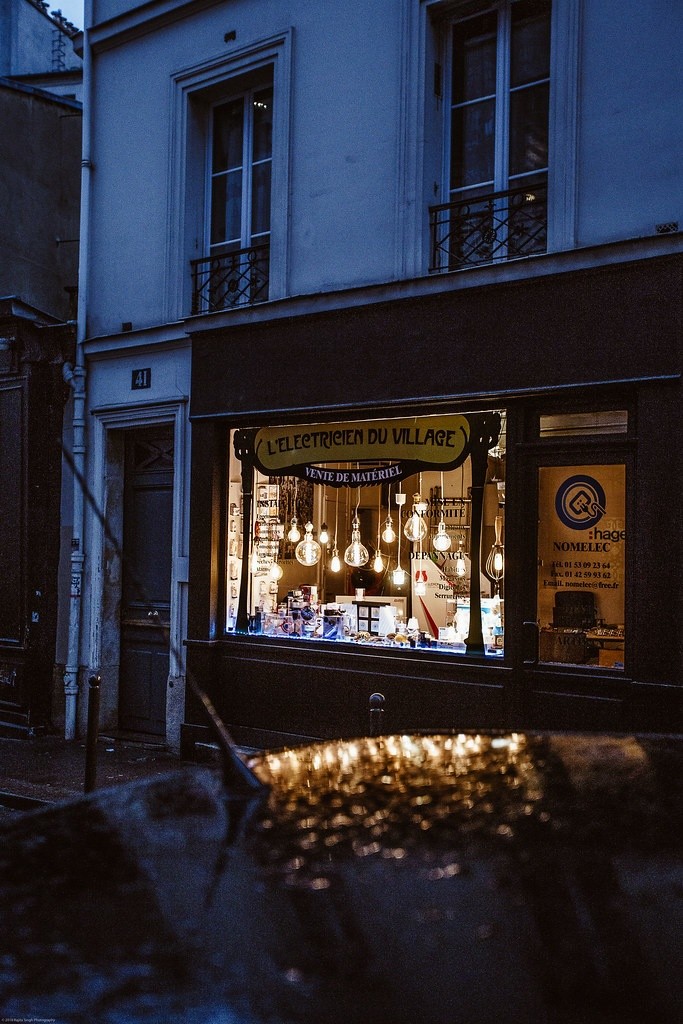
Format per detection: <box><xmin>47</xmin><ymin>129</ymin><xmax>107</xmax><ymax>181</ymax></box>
<box><xmin>0</xmin><ymin>729</ymin><xmax>682</xmax><ymax>1024</ymax></box>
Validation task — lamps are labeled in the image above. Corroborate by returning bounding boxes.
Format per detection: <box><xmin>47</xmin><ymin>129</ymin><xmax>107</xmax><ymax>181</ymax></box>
<box><xmin>381</xmin><ymin>483</ymin><xmax>397</xmax><ymax>543</ymax></box>
<box><xmin>415</xmin><ymin>472</ymin><xmax>426</xmax><ymax>595</ymax></box>
<box><xmin>373</xmin><ymin>485</ymin><xmax>384</xmax><ymax>572</ymax></box>
<box><xmin>318</xmin><ymin>522</ymin><xmax>329</xmax><ymax>544</ymax></box>
<box><xmin>343</xmin><ymin>486</ymin><xmax>369</xmax><ymax>568</ymax></box>
<box><xmin>433</xmin><ymin>471</ymin><xmax>452</xmax><ymax>551</ymax></box>
<box><xmin>294</xmin><ymin>519</ymin><xmax>322</xmax><ymax>567</ymax></box>
<box><xmin>330</xmin><ymin>488</ymin><xmax>342</xmax><ymax>573</ymax></box>
<box><xmin>393</xmin><ymin>493</ymin><xmax>406</xmax><ymax>585</ymax></box>
<box><xmin>0</xmin><ymin>335</ymin><xmax>25</xmax><ymax>352</ymax></box>
<box><xmin>287</xmin><ymin>477</ymin><xmax>301</xmax><ymax>542</ymax></box>
<box><xmin>454</xmin><ymin>465</ymin><xmax>466</xmax><ymax>576</ymax></box>
<box><xmin>404</xmin><ymin>492</ymin><xmax>428</xmax><ymax>543</ymax></box>
<box><xmin>485</xmin><ymin>516</ymin><xmax>505</xmax><ymax>598</ymax></box>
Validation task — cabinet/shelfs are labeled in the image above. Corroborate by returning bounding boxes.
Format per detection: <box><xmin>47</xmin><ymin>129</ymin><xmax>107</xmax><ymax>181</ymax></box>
<box><xmin>429</xmin><ymin>486</ymin><xmax>471</xmax><ymax>554</ymax></box>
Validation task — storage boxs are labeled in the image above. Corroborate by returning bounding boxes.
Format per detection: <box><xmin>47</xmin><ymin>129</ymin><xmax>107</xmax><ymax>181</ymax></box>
<box><xmin>302</xmin><ymin>586</ymin><xmax>318</xmax><ymax>595</ymax></box>
<box><xmin>303</xmin><ymin>594</ymin><xmax>318</xmax><ymax>603</ymax></box>
<box><xmin>327</xmin><ymin>602</ymin><xmax>356</xmax><ymax>631</ymax></box>
<box><xmin>465</xmin><ymin>481</ymin><xmax>504</xmax><ymax>575</ymax></box>
<box><xmin>598</xmin><ymin>650</ymin><xmax>625</xmax><ymax>668</ymax></box>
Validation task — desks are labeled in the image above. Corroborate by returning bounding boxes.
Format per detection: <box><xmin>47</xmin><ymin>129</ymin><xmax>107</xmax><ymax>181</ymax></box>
<box><xmin>546</xmin><ymin>626</ymin><xmax>624</xmax><ymax>649</ymax></box>
